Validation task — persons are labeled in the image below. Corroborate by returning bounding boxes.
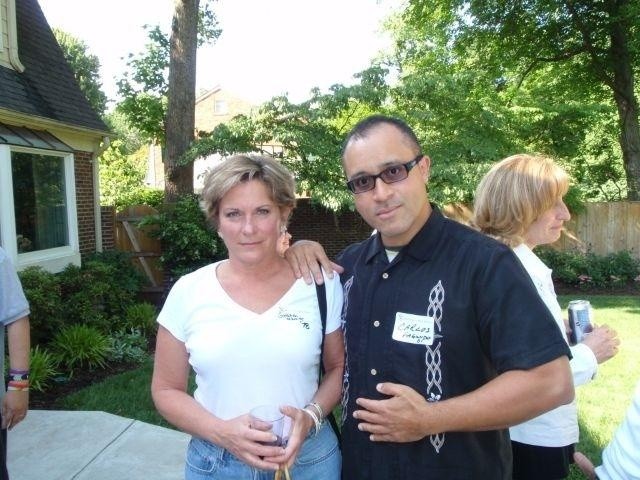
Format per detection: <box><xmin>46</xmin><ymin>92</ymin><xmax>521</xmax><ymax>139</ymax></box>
<box><xmin>283</xmin><ymin>115</ymin><xmax>578</xmax><ymax>480</ymax></box>
<box><xmin>276</xmin><ymin>225</ymin><xmax>293</xmax><ymax>257</ymax></box>
<box><xmin>572</xmin><ymin>380</ymin><xmax>638</xmax><ymax>480</ymax></box>
<box><xmin>0</xmin><ymin>245</ymin><xmax>32</xmax><ymax>479</ymax></box>
<box><xmin>150</xmin><ymin>151</ymin><xmax>348</xmax><ymax>480</ymax></box>
<box><xmin>473</xmin><ymin>153</ymin><xmax>624</xmax><ymax>479</ymax></box>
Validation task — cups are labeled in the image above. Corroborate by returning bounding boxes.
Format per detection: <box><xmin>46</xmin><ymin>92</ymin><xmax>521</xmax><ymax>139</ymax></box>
<box><xmin>250</xmin><ymin>405</ymin><xmax>286</xmax><ymax>460</ymax></box>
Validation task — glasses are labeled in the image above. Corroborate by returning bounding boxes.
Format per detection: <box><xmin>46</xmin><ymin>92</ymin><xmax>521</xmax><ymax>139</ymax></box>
<box><xmin>344</xmin><ymin>153</ymin><xmax>424</xmax><ymax>195</ymax></box>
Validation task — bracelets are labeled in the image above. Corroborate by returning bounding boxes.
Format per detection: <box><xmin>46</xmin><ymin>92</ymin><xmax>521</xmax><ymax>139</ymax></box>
<box><xmin>301</xmin><ymin>402</ymin><xmax>324</xmax><ymax>442</ymax></box>
<box><xmin>6</xmin><ymin>368</ymin><xmax>30</xmax><ymax>393</ymax></box>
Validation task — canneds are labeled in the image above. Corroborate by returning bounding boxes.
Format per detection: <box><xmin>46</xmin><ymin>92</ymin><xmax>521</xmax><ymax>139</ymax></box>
<box><xmin>568</xmin><ymin>300</ymin><xmax>594</xmax><ymax>346</ymax></box>
